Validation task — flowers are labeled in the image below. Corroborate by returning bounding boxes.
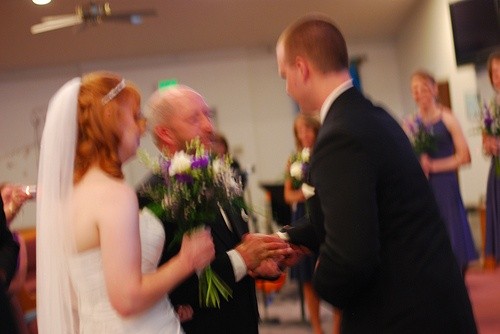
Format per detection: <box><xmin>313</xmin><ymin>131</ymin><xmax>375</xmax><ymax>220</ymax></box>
<box><xmin>139</xmin><ymin>136</ymin><xmax>265</xmax><ymax>308</ymax></box>
<box><xmin>468</xmin><ymin>93</ymin><xmax>500</xmax><ymax>180</ymax></box>
<box><xmin>404</xmin><ymin>113</ymin><xmax>436</xmax><ymax>179</ymax></box>
<box><xmin>284</xmin><ymin>147</ymin><xmax>315</xmax><ymax>219</ymax></box>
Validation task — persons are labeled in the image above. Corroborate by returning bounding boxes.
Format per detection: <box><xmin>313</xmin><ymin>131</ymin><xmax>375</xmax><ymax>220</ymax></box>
<box><xmin>275</xmin><ymin>14</ymin><xmax>478</xmax><ymax>334</ymax></box>
<box><xmin>481</xmin><ymin>53</ymin><xmax>500</xmax><ymax>265</ymax></box>
<box><xmin>35</xmin><ymin>74</ymin><xmax>215</xmax><ymax>334</ymax></box>
<box><xmin>284</xmin><ymin>115</ymin><xmax>340</xmax><ymax>334</ymax></box>
<box><xmin>137</xmin><ymin>84</ymin><xmax>292</xmax><ymax>334</ymax></box>
<box><xmin>403</xmin><ymin>72</ymin><xmax>479</xmax><ymax>264</ymax></box>
<box><xmin>0</xmin><ymin>184</ymin><xmax>36</xmax><ymax>334</ymax></box>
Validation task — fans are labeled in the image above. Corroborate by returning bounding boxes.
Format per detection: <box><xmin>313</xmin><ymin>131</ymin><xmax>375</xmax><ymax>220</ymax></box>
<box><xmin>30</xmin><ymin>1</ymin><xmax>158</xmax><ymax>33</ymax></box>
